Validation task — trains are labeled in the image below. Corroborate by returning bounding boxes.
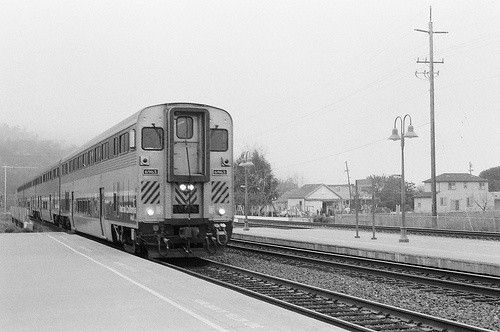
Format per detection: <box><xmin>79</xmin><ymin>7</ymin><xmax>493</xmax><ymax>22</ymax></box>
<box><xmin>12</xmin><ymin>101</ymin><xmax>237</xmax><ymax>261</ymax></box>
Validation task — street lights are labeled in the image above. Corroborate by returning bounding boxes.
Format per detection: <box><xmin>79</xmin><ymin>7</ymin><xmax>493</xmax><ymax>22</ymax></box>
<box><xmin>2</xmin><ymin>164</ymin><xmax>38</xmax><ymax>218</ymax></box>
<box><xmin>237</xmin><ymin>149</ymin><xmax>255</xmax><ymax>231</ymax></box>
<box><xmin>386</xmin><ymin>113</ymin><xmax>420</xmax><ymax>242</ymax></box>
